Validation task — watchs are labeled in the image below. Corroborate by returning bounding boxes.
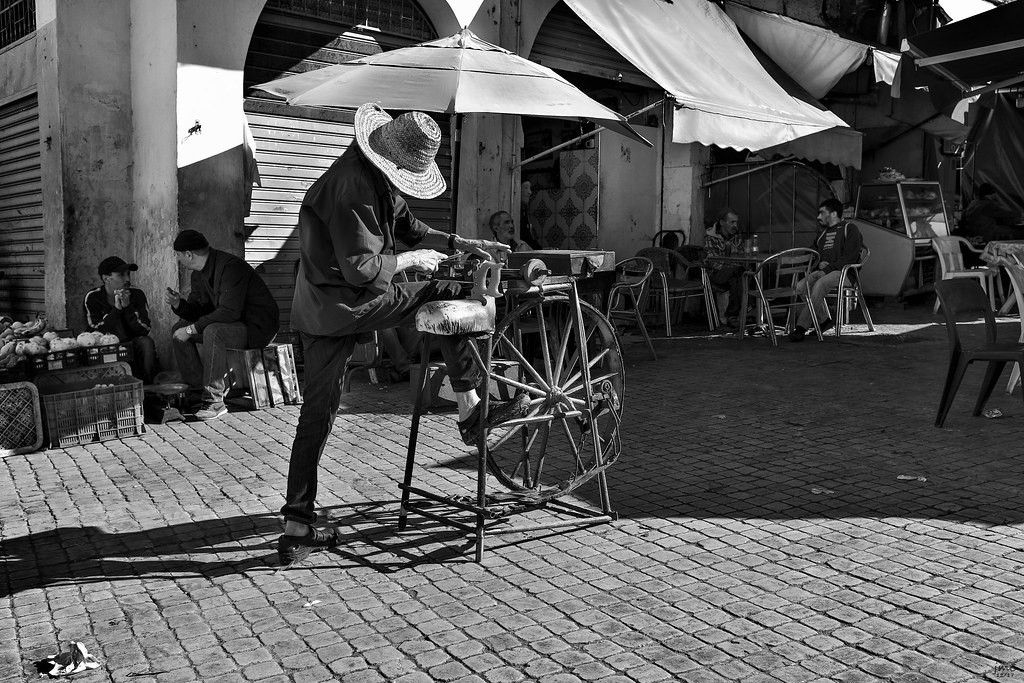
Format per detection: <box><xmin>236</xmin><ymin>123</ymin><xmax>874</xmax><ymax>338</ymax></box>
<box><xmin>185</xmin><ymin>325</ymin><xmax>197</xmax><ymax>337</ymax></box>
<box><xmin>448</xmin><ymin>234</ymin><xmax>460</xmax><ymax>251</ymax></box>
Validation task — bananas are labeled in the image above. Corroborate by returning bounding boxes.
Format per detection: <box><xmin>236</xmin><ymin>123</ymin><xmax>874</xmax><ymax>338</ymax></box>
<box><xmin>14</xmin><ymin>313</ymin><xmax>47</xmax><ymax>334</ymax></box>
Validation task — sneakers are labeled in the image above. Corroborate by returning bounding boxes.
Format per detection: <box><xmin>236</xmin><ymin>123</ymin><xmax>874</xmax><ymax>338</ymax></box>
<box><xmin>193</xmin><ymin>402</ymin><xmax>228</xmax><ymax>421</ymax></box>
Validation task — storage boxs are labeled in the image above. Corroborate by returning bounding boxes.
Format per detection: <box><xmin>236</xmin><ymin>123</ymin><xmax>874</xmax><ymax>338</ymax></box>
<box><xmin>0</xmin><ymin>382</ymin><xmax>43</xmax><ymax>457</ymax></box>
<box><xmin>28</xmin><ymin>341</ymin><xmax>134</xmax><ymax>384</ymax></box>
<box><xmin>39</xmin><ymin>375</ymin><xmax>146</xmax><ymax>450</ymax></box>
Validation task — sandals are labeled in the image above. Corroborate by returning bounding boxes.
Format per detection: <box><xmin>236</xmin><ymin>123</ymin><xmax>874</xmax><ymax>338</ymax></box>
<box><xmin>278</xmin><ymin>525</ymin><xmax>337</xmax><ymax>564</ymax></box>
<box><xmin>457</xmin><ymin>394</ymin><xmax>530</xmax><ymax>444</ymax></box>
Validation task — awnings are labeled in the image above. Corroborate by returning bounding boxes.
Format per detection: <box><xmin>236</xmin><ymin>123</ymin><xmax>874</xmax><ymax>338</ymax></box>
<box><xmin>891</xmin><ymin>0</ymin><xmax>1024</xmax><ymax>126</ymax></box>
<box><xmin>565</xmin><ymin>0</ymin><xmax>865</xmax><ymax>170</ymax></box>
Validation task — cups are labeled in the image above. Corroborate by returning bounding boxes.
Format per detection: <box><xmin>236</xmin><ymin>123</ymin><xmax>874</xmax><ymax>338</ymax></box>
<box><xmin>745</xmin><ymin>240</ymin><xmax>751</xmax><ymax>257</ymax></box>
<box><xmin>725</xmin><ymin>242</ymin><xmax>731</xmax><ymax>257</ymax></box>
<box><xmin>586</xmin><ymin>139</ymin><xmax>595</xmax><ymax>148</ymax></box>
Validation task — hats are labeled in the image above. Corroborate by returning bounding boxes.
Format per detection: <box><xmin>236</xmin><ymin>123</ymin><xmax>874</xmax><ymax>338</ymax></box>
<box><xmin>354</xmin><ymin>102</ymin><xmax>446</xmax><ymax>200</ymax></box>
<box><xmin>98</xmin><ymin>256</ymin><xmax>138</xmax><ymax>275</ymax></box>
<box><xmin>174</xmin><ymin>230</ymin><xmax>209</xmax><ymax>251</ymax></box>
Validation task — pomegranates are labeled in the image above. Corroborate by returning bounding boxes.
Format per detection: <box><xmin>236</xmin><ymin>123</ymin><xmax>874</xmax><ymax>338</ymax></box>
<box><xmin>0</xmin><ymin>331</ymin><xmax>118</xmax><ymax>358</ymax></box>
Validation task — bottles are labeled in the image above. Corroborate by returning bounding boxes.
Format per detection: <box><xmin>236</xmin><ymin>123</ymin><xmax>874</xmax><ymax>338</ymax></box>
<box><xmin>752</xmin><ymin>235</ymin><xmax>759</xmax><ymax>257</ymax></box>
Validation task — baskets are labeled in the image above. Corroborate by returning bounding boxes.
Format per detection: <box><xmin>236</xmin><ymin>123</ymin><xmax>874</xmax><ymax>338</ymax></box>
<box><xmin>26</xmin><ymin>342</ymin><xmax>134</xmax><ymax>373</ymax></box>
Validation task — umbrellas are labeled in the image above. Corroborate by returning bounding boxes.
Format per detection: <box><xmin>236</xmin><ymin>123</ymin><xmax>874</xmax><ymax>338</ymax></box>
<box><xmin>250</xmin><ymin>26</ymin><xmax>654</xmax><ymax>234</ymax></box>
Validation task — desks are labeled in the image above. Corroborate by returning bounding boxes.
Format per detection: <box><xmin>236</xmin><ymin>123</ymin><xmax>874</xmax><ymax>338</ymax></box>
<box><xmin>981</xmin><ymin>241</ymin><xmax>1024</xmax><ymax>343</ymax></box>
<box><xmin>710</xmin><ymin>254</ymin><xmax>799</xmax><ymax>332</ymax></box>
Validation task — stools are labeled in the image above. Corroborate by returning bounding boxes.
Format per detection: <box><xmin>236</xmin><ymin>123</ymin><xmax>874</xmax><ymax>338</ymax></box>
<box><xmin>225</xmin><ymin>342</ymin><xmax>299</xmax><ymax>409</ymax></box>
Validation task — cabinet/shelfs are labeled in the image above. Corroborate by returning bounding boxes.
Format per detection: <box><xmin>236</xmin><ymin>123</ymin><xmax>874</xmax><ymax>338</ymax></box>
<box><xmin>854</xmin><ymin>180</ymin><xmax>950</xmax><ymax>244</ymax></box>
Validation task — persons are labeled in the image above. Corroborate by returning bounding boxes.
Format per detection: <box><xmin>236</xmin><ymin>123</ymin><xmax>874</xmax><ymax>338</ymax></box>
<box><xmin>489</xmin><ymin>211</ymin><xmax>533</xmax><ymax>264</ymax></box>
<box><xmin>520</xmin><ymin>177</ymin><xmax>542</xmax><ymax>250</ymax></box>
<box><xmin>279</xmin><ymin>104</ymin><xmax>532</xmax><ymax>565</ymax></box>
<box><xmin>165</xmin><ymin>230</ymin><xmax>280</xmax><ymax>420</ymax></box>
<box><xmin>960</xmin><ymin>184</ymin><xmax>1024</xmax><ymax>301</ymax></box>
<box><xmin>381</xmin><ymin>324</ymin><xmax>424</xmax><ymax>384</ymax></box>
<box><xmin>788</xmin><ymin>198</ymin><xmax>863</xmax><ymax>341</ymax></box>
<box><xmin>83</xmin><ymin>256</ymin><xmax>161</xmax><ymax>412</ymax></box>
<box><xmin>704</xmin><ymin>209</ymin><xmax>753</xmax><ymax>325</ymax></box>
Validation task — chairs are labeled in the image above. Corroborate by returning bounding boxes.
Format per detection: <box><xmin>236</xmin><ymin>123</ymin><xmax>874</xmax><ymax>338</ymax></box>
<box><xmin>931</xmin><ymin>277</ymin><xmax>1023</xmax><ymax>427</ymax></box>
<box><xmin>739</xmin><ymin>247</ymin><xmax>824</xmax><ymax>347</ymax></box>
<box><xmin>929</xmin><ymin>236</ymin><xmax>1005</xmax><ymax>316</ymax></box>
<box><xmin>635</xmin><ymin>249</ymin><xmax>715</xmax><ymax>338</ymax></box>
<box><xmin>600</xmin><ymin>256</ymin><xmax>656</xmax><ymax>368</ymax></box>
<box><xmin>808</xmin><ymin>245</ymin><xmax>876</xmax><ymax>338</ymax></box>
<box><xmin>674</xmin><ymin>245</ymin><xmax>722</xmax><ymax>328</ymax></box>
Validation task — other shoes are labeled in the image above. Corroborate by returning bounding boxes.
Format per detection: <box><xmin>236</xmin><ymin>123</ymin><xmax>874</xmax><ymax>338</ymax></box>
<box><xmin>809</xmin><ymin>320</ymin><xmax>834</xmax><ymax>336</ymax></box>
<box><xmin>727</xmin><ymin>316</ymin><xmax>739</xmax><ymax>328</ymax></box>
<box><xmin>787</xmin><ymin>332</ymin><xmax>805</xmax><ymax>342</ymax></box>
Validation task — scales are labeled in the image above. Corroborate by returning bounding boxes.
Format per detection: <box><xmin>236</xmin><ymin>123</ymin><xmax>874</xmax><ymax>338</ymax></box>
<box><xmin>147</xmin><ymin>383</ymin><xmax>188</xmax><ymax>424</ymax></box>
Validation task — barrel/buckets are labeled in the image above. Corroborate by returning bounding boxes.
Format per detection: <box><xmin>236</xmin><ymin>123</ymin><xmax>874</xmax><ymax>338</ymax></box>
<box><xmin>832</xmin><ymin>180</ymin><xmax>850</xmax><ymax>203</ymax></box>
